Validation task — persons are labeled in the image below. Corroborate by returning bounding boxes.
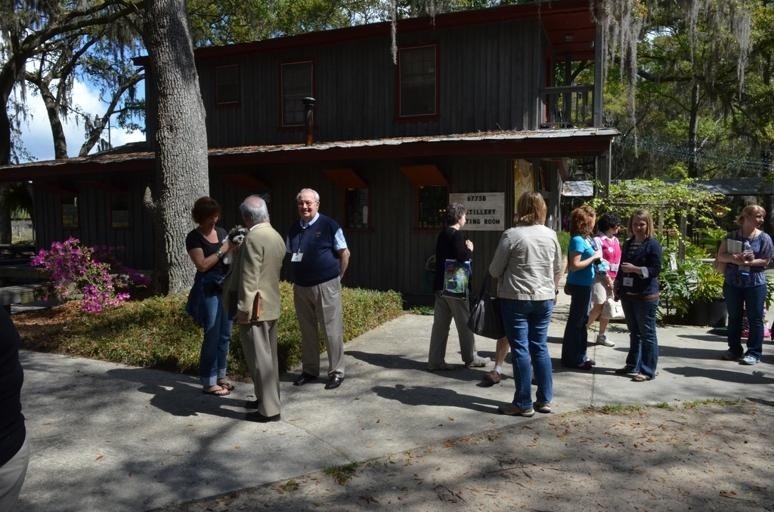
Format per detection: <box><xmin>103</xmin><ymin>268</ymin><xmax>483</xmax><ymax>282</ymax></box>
<box><xmin>186</xmin><ymin>195</ymin><xmax>250</xmax><ymax>395</ymax></box>
<box><xmin>485</xmin><ymin>337</ymin><xmax>511</xmax><ymax>384</ymax></box>
<box><xmin>426</xmin><ymin>202</ymin><xmax>490</xmax><ymax>372</ymax></box>
<box><xmin>0</xmin><ymin>304</ymin><xmax>30</xmax><ymax>512</ymax></box>
<box><xmin>285</xmin><ymin>188</ymin><xmax>350</xmax><ymax>389</ymax></box>
<box><xmin>586</xmin><ymin>211</ymin><xmax>621</xmax><ymax>348</ymax></box>
<box><xmin>223</xmin><ymin>196</ymin><xmax>287</xmax><ymax>422</ymax></box>
<box><xmin>564</xmin><ymin>205</ymin><xmax>602</xmax><ymax>372</ymax></box>
<box><xmin>716</xmin><ymin>204</ymin><xmax>774</xmax><ymax>366</ymax></box>
<box><xmin>613</xmin><ymin>208</ymin><xmax>664</xmax><ymax>383</ymax></box>
<box><xmin>489</xmin><ymin>191</ymin><xmax>562</xmax><ymax>417</ymax></box>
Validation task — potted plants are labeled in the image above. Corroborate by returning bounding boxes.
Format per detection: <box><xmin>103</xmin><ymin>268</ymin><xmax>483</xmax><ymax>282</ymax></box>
<box><xmin>661</xmin><ymin>264</ymin><xmax>727</xmax><ymax>326</ymax></box>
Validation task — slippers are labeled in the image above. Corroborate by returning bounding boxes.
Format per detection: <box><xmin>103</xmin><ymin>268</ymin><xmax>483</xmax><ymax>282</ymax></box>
<box><xmin>204</xmin><ymin>387</ymin><xmax>230</xmax><ymax>396</ymax></box>
<box><xmin>217</xmin><ymin>383</ymin><xmax>234</xmax><ymax>391</ymax></box>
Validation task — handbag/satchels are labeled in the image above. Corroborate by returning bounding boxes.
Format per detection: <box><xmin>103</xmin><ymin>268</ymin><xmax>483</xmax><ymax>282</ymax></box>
<box><xmin>468</xmin><ymin>296</ymin><xmax>506</xmax><ymax>339</ymax></box>
<box><xmin>442</xmin><ymin>259</ymin><xmax>470</xmax><ymax>299</ymax></box>
<box><xmin>227</xmin><ymin>291</ymin><xmax>261</xmax><ymax>320</ymax></box>
<box><xmin>601</xmin><ymin>297</ymin><xmax>625</xmax><ymax>321</ymax></box>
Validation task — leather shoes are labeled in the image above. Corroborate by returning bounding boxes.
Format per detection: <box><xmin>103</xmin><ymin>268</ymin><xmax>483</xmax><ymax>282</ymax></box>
<box><xmin>246</xmin><ymin>401</ymin><xmax>258</xmax><ymax>409</ymax></box>
<box><xmin>584</xmin><ymin>359</ymin><xmax>595</xmax><ymax>365</ymax></box>
<box><xmin>570</xmin><ymin>362</ymin><xmax>592</xmax><ymax>370</ymax></box>
<box><xmin>246</xmin><ymin>411</ymin><xmax>280</xmax><ymax>422</ymax></box>
<box><xmin>325</xmin><ymin>373</ymin><xmax>344</xmax><ymax>389</ymax></box>
<box><xmin>296</xmin><ymin>374</ymin><xmax>317</xmax><ymax>386</ymax></box>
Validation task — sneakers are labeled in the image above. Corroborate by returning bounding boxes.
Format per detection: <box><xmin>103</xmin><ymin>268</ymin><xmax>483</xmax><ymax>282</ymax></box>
<box><xmin>633</xmin><ymin>373</ymin><xmax>651</xmax><ymax>382</ymax></box>
<box><xmin>465</xmin><ymin>358</ymin><xmax>490</xmax><ymax>367</ymax></box>
<box><xmin>427</xmin><ymin>362</ymin><xmax>456</xmax><ymax>371</ymax></box>
<box><xmin>483</xmin><ymin>371</ymin><xmax>501</xmax><ymax>384</ymax></box>
<box><xmin>740</xmin><ymin>354</ymin><xmax>760</xmax><ymax>365</ymax></box>
<box><xmin>533</xmin><ymin>402</ymin><xmax>551</xmax><ymax>413</ymax></box>
<box><xmin>499</xmin><ymin>402</ymin><xmax>535</xmax><ymax>416</ymax></box>
<box><xmin>615</xmin><ymin>366</ymin><xmax>640</xmax><ymax>375</ymax></box>
<box><xmin>722</xmin><ymin>351</ymin><xmax>744</xmax><ymax>360</ymax></box>
<box><xmin>715</xmin><ymin>260</ymin><xmax>725</xmax><ymax>272</ymax></box>
<box><xmin>597</xmin><ymin>335</ymin><xmax>615</xmax><ymax>347</ymax></box>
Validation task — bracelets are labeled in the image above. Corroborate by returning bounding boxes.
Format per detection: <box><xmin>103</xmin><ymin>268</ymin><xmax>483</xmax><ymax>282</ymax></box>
<box><xmin>216</xmin><ymin>248</ymin><xmax>225</xmax><ymax>259</ymax></box>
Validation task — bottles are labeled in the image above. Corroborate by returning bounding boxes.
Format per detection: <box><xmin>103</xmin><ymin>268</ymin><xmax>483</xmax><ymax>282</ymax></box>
<box><xmin>743</xmin><ymin>240</ymin><xmax>753</xmax><ymax>264</ymax></box>
